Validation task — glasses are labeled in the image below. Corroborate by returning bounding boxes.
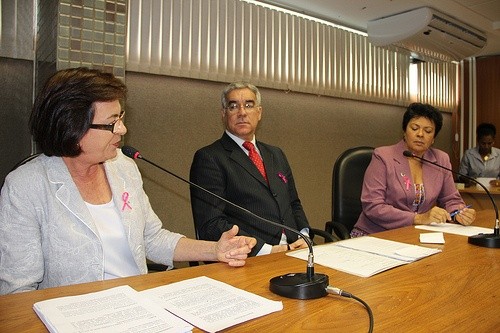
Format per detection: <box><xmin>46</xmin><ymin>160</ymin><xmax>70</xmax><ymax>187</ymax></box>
<box><xmin>225</xmin><ymin>102</ymin><xmax>257</xmax><ymax>113</ymax></box>
<box><xmin>90</xmin><ymin>111</ymin><xmax>125</xmax><ymax>134</ymax></box>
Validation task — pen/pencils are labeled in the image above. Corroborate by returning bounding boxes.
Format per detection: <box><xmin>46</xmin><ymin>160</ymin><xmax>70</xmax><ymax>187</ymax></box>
<box><xmin>451</xmin><ymin>204</ymin><xmax>472</xmax><ymax>217</ymax></box>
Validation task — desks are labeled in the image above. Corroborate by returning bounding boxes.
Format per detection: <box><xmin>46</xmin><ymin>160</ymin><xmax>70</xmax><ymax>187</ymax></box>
<box><xmin>1</xmin><ymin>209</ymin><xmax>500</xmax><ymax>333</ymax></box>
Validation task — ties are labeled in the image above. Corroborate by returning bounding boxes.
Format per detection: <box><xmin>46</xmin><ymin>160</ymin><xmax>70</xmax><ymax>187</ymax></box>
<box><xmin>242</xmin><ymin>140</ymin><xmax>269</xmax><ymax>185</ymax></box>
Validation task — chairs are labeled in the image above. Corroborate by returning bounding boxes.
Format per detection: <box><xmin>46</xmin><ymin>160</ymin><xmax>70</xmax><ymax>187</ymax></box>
<box><xmin>324</xmin><ymin>146</ymin><xmax>375</xmax><ymax>243</ymax></box>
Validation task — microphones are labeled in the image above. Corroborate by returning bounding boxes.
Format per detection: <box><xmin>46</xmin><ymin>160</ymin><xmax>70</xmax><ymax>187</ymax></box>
<box><xmin>121</xmin><ymin>144</ymin><xmax>330</xmax><ymax>301</ymax></box>
<box><xmin>402</xmin><ymin>150</ymin><xmax>500</xmax><ymax>250</ymax></box>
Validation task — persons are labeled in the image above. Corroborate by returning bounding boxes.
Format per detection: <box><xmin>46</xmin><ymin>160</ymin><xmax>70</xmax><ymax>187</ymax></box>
<box><xmin>0</xmin><ymin>68</ymin><xmax>258</xmax><ymax>296</ymax></box>
<box><xmin>454</xmin><ymin>123</ymin><xmax>500</xmax><ymax>187</ymax></box>
<box><xmin>188</xmin><ymin>81</ymin><xmax>315</xmax><ymax>263</ymax></box>
<box><xmin>349</xmin><ymin>102</ymin><xmax>476</xmax><ymax>238</ymax></box>
